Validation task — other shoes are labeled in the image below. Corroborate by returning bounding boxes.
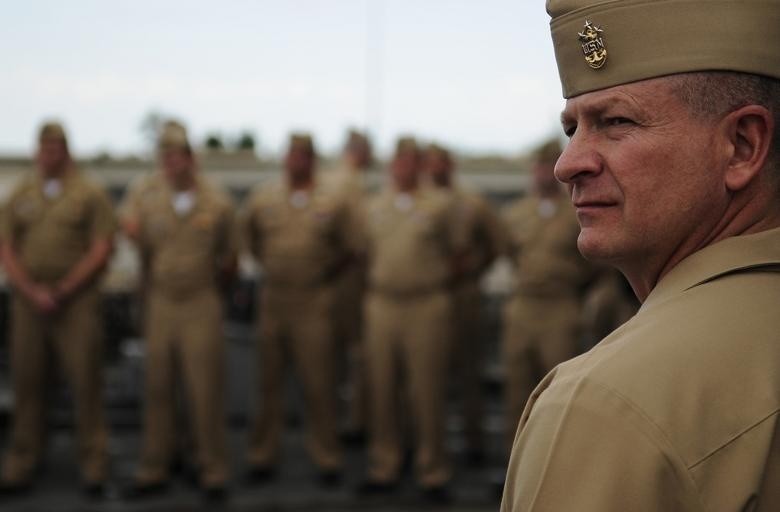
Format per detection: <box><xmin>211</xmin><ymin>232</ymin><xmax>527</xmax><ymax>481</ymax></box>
<box><xmin>237</xmin><ymin>459</ymin><xmax>280</xmax><ymax>488</ymax></box>
<box><xmin>194</xmin><ymin>465</ymin><xmax>228</xmax><ymax>499</ymax></box>
<box><xmin>314</xmin><ymin>461</ymin><xmax>346</xmax><ymax>487</ymax></box>
<box><xmin>474</xmin><ymin>442</ymin><xmax>508</xmax><ymax>465</ymax></box>
<box><xmin>81</xmin><ymin>464</ymin><xmax>105</xmax><ymax>497</ymax></box>
<box><xmin>354</xmin><ymin>467</ymin><xmax>405</xmax><ymax>498</ymax></box>
<box><xmin>118</xmin><ymin>471</ymin><xmax>170</xmax><ymax>498</ymax></box>
<box><xmin>421</xmin><ymin>482</ymin><xmax>452</xmax><ymax>498</ymax></box>
<box><xmin>0</xmin><ymin>460</ymin><xmax>46</xmax><ymax>499</ymax></box>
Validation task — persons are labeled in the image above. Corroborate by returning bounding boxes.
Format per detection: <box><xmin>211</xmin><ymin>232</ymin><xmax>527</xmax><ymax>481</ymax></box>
<box><xmin>113</xmin><ymin>119</ymin><xmax>247</xmax><ymax>492</ymax></box>
<box><xmin>1</xmin><ymin>121</ymin><xmax>120</xmax><ymax>492</ymax></box>
<box><xmin>423</xmin><ymin>141</ymin><xmax>500</xmax><ymax>462</ymax></box>
<box><xmin>340</xmin><ymin>126</ymin><xmax>402</xmax><ymax>442</ymax></box>
<box><xmin>235</xmin><ymin>133</ymin><xmax>364</xmax><ymax>479</ymax></box>
<box><xmin>484</xmin><ymin>134</ymin><xmax>619</xmax><ymax>499</ymax></box>
<box><xmin>500</xmin><ymin>2</ymin><xmax>779</xmax><ymax>511</ymax></box>
<box><xmin>356</xmin><ymin>135</ymin><xmax>483</xmax><ymax>491</ymax></box>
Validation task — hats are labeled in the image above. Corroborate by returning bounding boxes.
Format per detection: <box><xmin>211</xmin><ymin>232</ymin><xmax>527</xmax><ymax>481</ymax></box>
<box><xmin>162</xmin><ymin>124</ymin><xmax>188</xmax><ymax>147</ymax></box>
<box><xmin>534</xmin><ymin>141</ymin><xmax>561</xmax><ymax>162</ymax></box>
<box><xmin>40</xmin><ymin>125</ymin><xmax>63</xmax><ymax>141</ymax></box>
<box><xmin>427</xmin><ymin>144</ymin><xmax>447</xmax><ymax>162</ymax></box>
<box><xmin>398</xmin><ymin>139</ymin><xmax>417</xmax><ymax>155</ymax></box>
<box><xmin>292</xmin><ymin>135</ymin><xmax>312</xmax><ymax>152</ymax></box>
<box><xmin>349</xmin><ymin>131</ymin><xmax>368</xmax><ymax>148</ymax></box>
<box><xmin>545</xmin><ymin>0</ymin><xmax>780</xmax><ymax>99</ymax></box>
